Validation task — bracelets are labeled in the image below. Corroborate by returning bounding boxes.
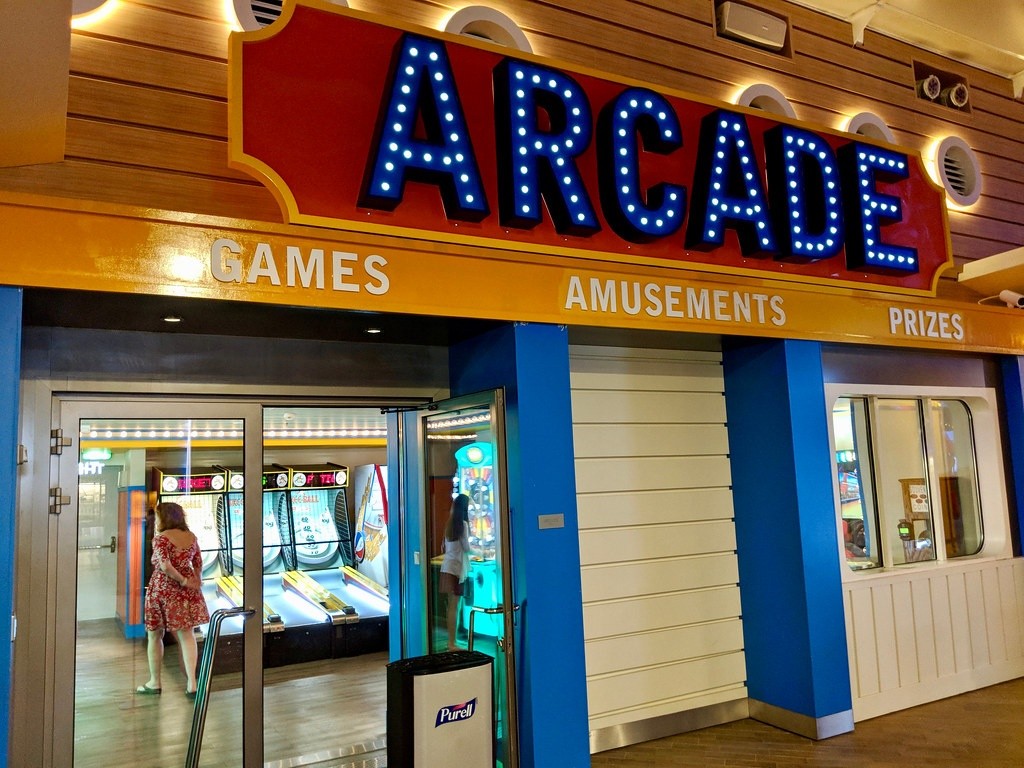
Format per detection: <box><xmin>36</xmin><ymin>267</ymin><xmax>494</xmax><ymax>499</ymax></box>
<box><xmin>180</xmin><ymin>578</ymin><xmax>187</xmax><ymax>586</ymax></box>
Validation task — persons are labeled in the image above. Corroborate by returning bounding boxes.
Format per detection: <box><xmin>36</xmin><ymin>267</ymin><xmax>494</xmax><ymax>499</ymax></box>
<box><xmin>136</xmin><ymin>502</ymin><xmax>210</xmax><ymax>699</ymax></box>
<box><xmin>438</xmin><ymin>494</ymin><xmax>498</xmax><ymax>652</ymax></box>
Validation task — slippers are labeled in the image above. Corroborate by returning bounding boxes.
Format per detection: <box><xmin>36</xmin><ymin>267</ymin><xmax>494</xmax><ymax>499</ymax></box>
<box><xmin>131</xmin><ymin>684</ymin><xmax>162</xmax><ymax>695</ymax></box>
<box><xmin>184</xmin><ymin>688</ymin><xmax>197</xmax><ymax>699</ymax></box>
